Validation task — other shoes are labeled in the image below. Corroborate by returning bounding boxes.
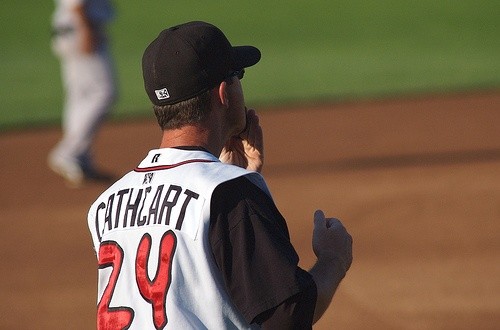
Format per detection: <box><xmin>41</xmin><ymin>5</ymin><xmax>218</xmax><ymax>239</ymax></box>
<box><xmin>47</xmin><ymin>150</ymin><xmax>85</xmax><ymax>183</ymax></box>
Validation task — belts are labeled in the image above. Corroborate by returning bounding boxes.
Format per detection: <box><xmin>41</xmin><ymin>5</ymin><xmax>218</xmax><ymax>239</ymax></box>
<box><xmin>49</xmin><ymin>26</ymin><xmax>75</xmax><ymax>37</ymax></box>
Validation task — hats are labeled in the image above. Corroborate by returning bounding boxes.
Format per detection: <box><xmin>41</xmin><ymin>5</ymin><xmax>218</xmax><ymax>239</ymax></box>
<box><xmin>141</xmin><ymin>20</ymin><xmax>262</xmax><ymax>107</ymax></box>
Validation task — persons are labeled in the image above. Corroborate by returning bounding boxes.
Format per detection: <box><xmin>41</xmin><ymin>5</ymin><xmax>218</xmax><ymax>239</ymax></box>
<box><xmin>87</xmin><ymin>20</ymin><xmax>354</xmax><ymax>330</ymax></box>
<box><xmin>47</xmin><ymin>0</ymin><xmax>119</xmax><ymax>182</ymax></box>
